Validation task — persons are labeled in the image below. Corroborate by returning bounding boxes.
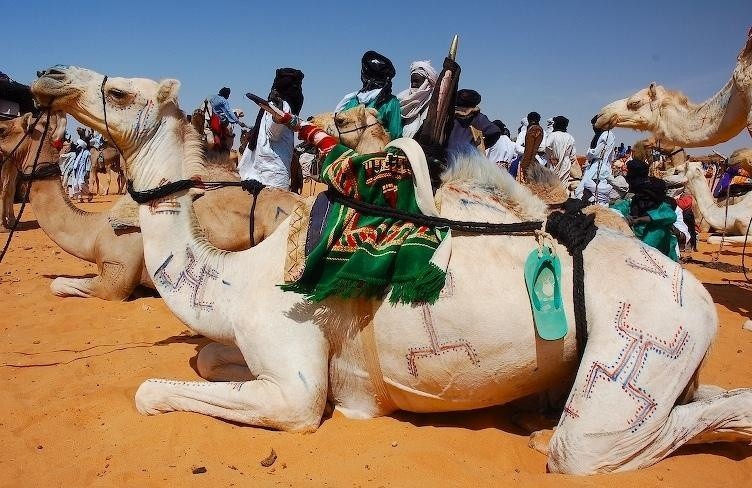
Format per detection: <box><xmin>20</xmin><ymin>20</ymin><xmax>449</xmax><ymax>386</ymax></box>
<box><xmin>485</xmin><ymin>120</ymin><xmax>520</xmax><ymax>179</ymax></box>
<box><xmin>574</xmin><ymin>115</ymin><xmax>620</xmax><ymax>206</ymax></box>
<box><xmin>443</xmin><ymin>90</ymin><xmax>500</xmax><ymax>166</ymax></box>
<box><xmin>521</xmin><ymin>112</ymin><xmax>546</xmax><ymax>183</ymax></box>
<box><xmin>398</xmin><ymin>61</ymin><xmax>439</xmax><ymax>139</ymax></box>
<box><xmin>515</xmin><ymin>118</ymin><xmax>554</xmax><ymax>154</ymax></box>
<box><xmin>59</xmin><ymin>130</ymin><xmax>102</xmax><ymax>202</ymax></box>
<box><xmin>544</xmin><ymin>116</ymin><xmax>576</xmax><ymax>191</ymax></box>
<box><xmin>612</xmin><ymin>160</ymin><xmax>682</xmax><ymax>262</ymax></box>
<box><xmin>614</xmin><ymin>143</ymin><xmax>631</xmax><ymax>158</ymax></box>
<box><xmin>201</xmin><ymin>87</ymin><xmax>244</xmax><ymax>128</ymax></box>
<box><xmin>239</xmin><ymin>68</ymin><xmax>303</xmax><ymax>190</ymax></box>
<box><xmin>336</xmin><ymin>51</ymin><xmax>403</xmax><ymax>139</ymax></box>
<box><xmin>299</xmin><ymin>117</ymin><xmax>317</xmax><ymax>184</ymax></box>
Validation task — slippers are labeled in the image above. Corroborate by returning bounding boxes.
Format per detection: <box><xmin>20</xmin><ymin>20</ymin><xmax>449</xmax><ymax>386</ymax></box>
<box><xmin>523</xmin><ymin>247</ymin><xmax>568</xmax><ymax>341</ymax></box>
